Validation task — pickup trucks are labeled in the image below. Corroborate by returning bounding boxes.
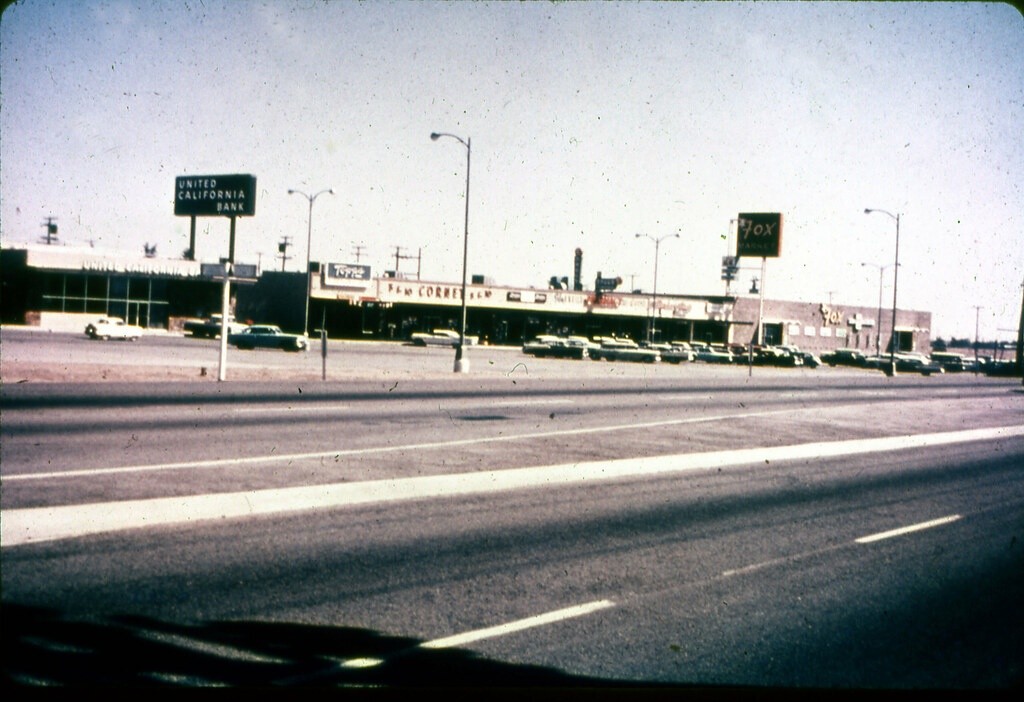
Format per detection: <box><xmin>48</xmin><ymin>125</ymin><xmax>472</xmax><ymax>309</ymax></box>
<box><xmin>183</xmin><ymin>313</ymin><xmax>251</xmax><ymax>339</ymax></box>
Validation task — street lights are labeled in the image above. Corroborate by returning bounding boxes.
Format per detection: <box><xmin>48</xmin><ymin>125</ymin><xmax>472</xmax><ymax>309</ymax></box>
<box><xmin>634</xmin><ymin>233</ymin><xmax>680</xmax><ymax>347</ymax></box>
<box><xmin>428</xmin><ymin>130</ymin><xmax>473</xmax><ymax>373</ymax></box>
<box><xmin>288</xmin><ymin>187</ymin><xmax>335</xmax><ymax>337</ymax></box>
<box><xmin>865</xmin><ymin>208</ymin><xmax>900</xmax><ymax>376</ymax></box>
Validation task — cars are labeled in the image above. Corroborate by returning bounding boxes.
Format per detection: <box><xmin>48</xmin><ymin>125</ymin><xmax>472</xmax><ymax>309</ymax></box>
<box><xmin>228</xmin><ymin>323</ymin><xmax>305</xmax><ymax>352</ymax></box>
<box><xmin>85</xmin><ymin>317</ymin><xmax>143</xmax><ymax>342</ymax></box>
<box><xmin>412</xmin><ymin>328</ymin><xmax>480</xmax><ymax>348</ymax></box>
<box><xmin>523</xmin><ymin>333</ymin><xmax>1005</xmax><ymax>375</ymax></box>
